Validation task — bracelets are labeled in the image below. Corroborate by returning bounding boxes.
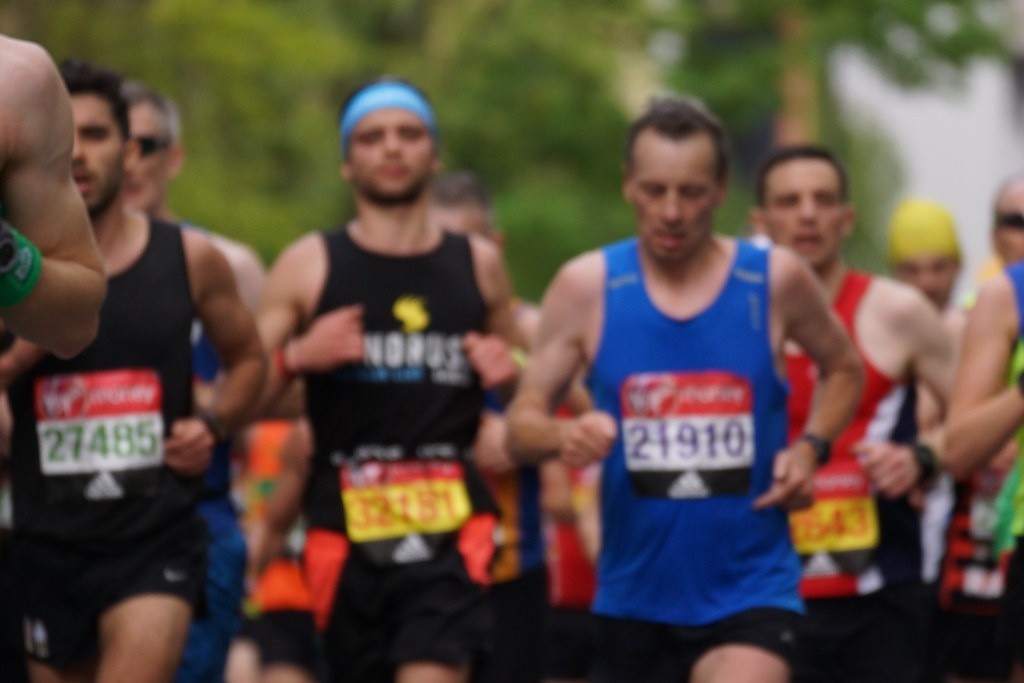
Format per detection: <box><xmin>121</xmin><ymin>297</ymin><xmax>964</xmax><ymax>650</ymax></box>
<box><xmin>1018</xmin><ymin>372</ymin><xmax>1024</xmax><ymax>393</ymax></box>
<box><xmin>275</xmin><ymin>344</ymin><xmax>296</xmax><ymax>380</ymax></box>
<box><xmin>0</xmin><ymin>227</ymin><xmax>42</xmax><ymax>307</ymax></box>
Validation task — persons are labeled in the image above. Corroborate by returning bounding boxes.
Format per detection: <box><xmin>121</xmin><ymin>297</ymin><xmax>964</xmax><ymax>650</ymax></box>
<box><xmin>756</xmin><ymin>143</ymin><xmax>959</xmax><ymax>683</ymax></box>
<box><xmin>1</xmin><ymin>67</ymin><xmax>267</xmax><ymax>683</ymax></box>
<box><xmin>503</xmin><ymin>101</ymin><xmax>865</xmax><ymax>683</ymax></box>
<box><xmin>225</xmin><ymin>77</ymin><xmax>603</xmax><ymax>683</ymax></box>
<box><xmin>122</xmin><ymin>86</ymin><xmax>269</xmax><ymax>683</ymax></box>
<box><xmin>889</xmin><ymin>177</ymin><xmax>1024</xmax><ymax>683</ymax></box>
<box><xmin>0</xmin><ymin>35</ymin><xmax>107</xmax><ymax>683</ymax></box>
<box><xmin>945</xmin><ymin>262</ymin><xmax>1024</xmax><ymax>683</ymax></box>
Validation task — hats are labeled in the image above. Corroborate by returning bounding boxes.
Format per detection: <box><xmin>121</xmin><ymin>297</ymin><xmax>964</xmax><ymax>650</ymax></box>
<box><xmin>889</xmin><ymin>202</ymin><xmax>959</xmax><ymax>272</ymax></box>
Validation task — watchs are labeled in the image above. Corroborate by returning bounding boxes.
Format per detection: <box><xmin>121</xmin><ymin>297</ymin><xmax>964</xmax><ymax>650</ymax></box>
<box><xmin>910</xmin><ymin>442</ymin><xmax>935</xmax><ymax>484</ymax></box>
<box><xmin>805</xmin><ymin>435</ymin><xmax>832</xmax><ymax>464</ymax></box>
<box><xmin>201</xmin><ymin>412</ymin><xmax>226</xmax><ymax>440</ymax></box>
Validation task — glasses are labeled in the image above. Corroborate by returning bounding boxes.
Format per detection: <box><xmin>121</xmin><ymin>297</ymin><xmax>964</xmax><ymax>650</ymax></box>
<box><xmin>137</xmin><ymin>138</ymin><xmax>168</xmax><ymax>155</ymax></box>
<box><xmin>998</xmin><ymin>214</ymin><xmax>1024</xmax><ymax>230</ymax></box>
